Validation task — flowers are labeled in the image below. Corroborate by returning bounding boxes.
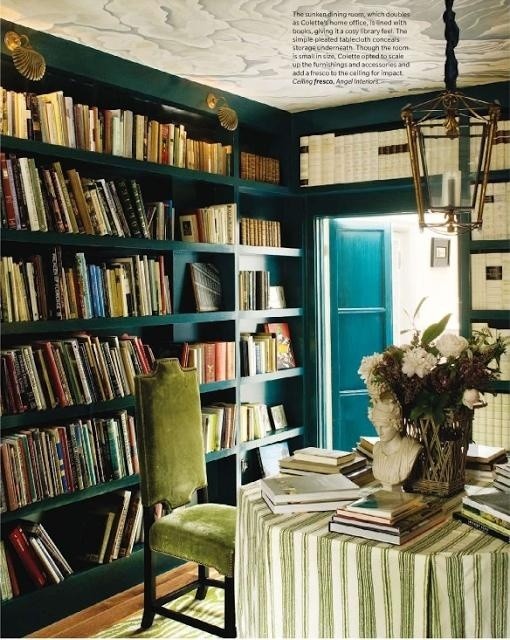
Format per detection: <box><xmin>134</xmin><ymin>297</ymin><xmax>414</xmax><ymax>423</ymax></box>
<box><xmin>358</xmin><ymin>295</ymin><xmax>510</xmax><ymax>490</ymax></box>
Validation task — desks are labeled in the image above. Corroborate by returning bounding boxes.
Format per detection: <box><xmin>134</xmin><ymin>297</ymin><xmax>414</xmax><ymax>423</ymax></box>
<box><xmin>235</xmin><ymin>463</ymin><xmax>510</xmax><ymax>638</ymax></box>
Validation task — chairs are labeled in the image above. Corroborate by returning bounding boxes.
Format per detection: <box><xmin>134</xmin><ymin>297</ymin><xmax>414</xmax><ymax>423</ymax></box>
<box><xmin>132</xmin><ymin>356</ymin><xmax>237</xmax><ymax>638</ymax></box>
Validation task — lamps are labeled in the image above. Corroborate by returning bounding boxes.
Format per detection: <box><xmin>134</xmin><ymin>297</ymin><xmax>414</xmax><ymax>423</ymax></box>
<box><xmin>402</xmin><ymin>0</ymin><xmax>501</xmax><ymax>236</ymax></box>
<box><xmin>207</xmin><ymin>93</ymin><xmax>238</xmax><ymax>131</ymax></box>
<box><xmin>4</xmin><ymin>31</ymin><xmax>47</xmax><ymax>80</ymax></box>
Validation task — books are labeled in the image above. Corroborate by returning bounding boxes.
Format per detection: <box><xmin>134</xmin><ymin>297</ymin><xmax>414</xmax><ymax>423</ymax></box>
<box><xmin>257</xmin><ymin>427</ymin><xmax>510</xmax><ymax>546</ymax></box>
<box><xmin>1</xmin><ymin>85</ymin><xmax>304</xmax><ymax>605</ymax></box>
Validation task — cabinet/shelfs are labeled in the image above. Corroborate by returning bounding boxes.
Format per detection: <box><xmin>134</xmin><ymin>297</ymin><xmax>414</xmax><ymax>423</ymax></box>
<box><xmin>293</xmin><ymin>78</ymin><xmax>510</xmax><ymax>451</ymax></box>
<box><xmin>0</xmin><ymin>18</ymin><xmax>310</xmax><ymax>638</ymax></box>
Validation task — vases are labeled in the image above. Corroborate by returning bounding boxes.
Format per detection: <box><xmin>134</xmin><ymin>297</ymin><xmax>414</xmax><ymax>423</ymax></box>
<box><xmin>406</xmin><ymin>409</ymin><xmax>465</xmax><ymax>497</ymax></box>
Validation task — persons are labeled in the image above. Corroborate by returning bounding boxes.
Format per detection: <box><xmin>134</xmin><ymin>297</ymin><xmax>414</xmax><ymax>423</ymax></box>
<box><xmin>362</xmin><ymin>399</ymin><xmax>424</xmax><ymax>485</ymax></box>
